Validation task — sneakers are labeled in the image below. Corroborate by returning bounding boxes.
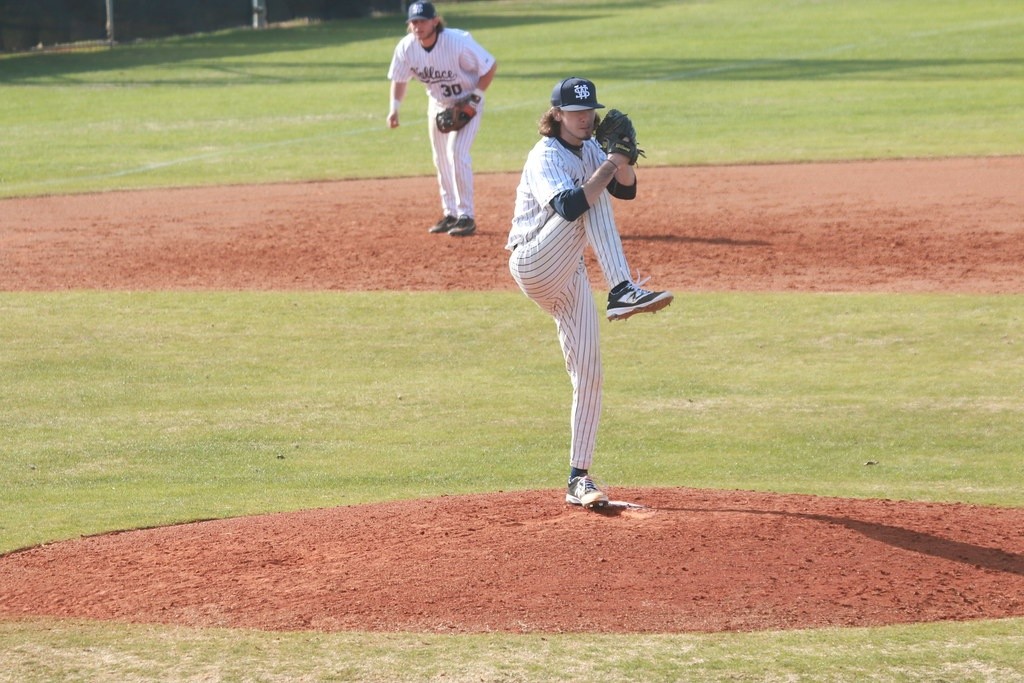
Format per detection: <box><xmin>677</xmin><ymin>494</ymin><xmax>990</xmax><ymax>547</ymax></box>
<box><xmin>448</xmin><ymin>218</ymin><xmax>476</xmax><ymax>235</ymax></box>
<box><xmin>605</xmin><ymin>269</ymin><xmax>673</xmax><ymax>321</ymax></box>
<box><xmin>566</xmin><ymin>475</ymin><xmax>609</xmax><ymax>510</ymax></box>
<box><xmin>430</xmin><ymin>216</ymin><xmax>457</xmax><ymax>233</ymax></box>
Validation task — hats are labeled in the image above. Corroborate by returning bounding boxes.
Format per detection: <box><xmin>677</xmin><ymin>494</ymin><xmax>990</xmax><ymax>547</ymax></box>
<box><xmin>405</xmin><ymin>1</ymin><xmax>435</xmax><ymax>22</ymax></box>
<box><xmin>551</xmin><ymin>76</ymin><xmax>606</xmax><ymax>111</ymax></box>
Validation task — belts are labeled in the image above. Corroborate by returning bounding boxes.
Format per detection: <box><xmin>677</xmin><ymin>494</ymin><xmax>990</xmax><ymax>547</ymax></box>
<box><xmin>513</xmin><ymin>245</ymin><xmax>517</xmax><ymax>249</ymax></box>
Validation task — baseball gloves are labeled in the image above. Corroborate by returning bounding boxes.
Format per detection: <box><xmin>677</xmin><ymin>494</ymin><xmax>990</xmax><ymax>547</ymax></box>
<box><xmin>595</xmin><ymin>108</ymin><xmax>639</xmax><ymax>167</ymax></box>
<box><xmin>436</xmin><ymin>101</ymin><xmax>477</xmax><ymax>133</ymax></box>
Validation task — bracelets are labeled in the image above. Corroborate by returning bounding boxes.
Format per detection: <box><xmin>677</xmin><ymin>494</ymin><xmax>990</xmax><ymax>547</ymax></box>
<box><xmin>388</xmin><ymin>99</ymin><xmax>402</xmax><ymax>112</ymax></box>
<box><xmin>468</xmin><ymin>89</ymin><xmax>484</xmax><ymax>108</ymax></box>
<box><xmin>607</xmin><ymin>159</ymin><xmax>619</xmax><ymax>172</ymax></box>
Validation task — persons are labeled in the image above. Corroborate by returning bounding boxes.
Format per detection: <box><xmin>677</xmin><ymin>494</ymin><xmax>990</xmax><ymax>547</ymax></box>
<box><xmin>386</xmin><ymin>0</ymin><xmax>497</xmax><ymax>235</ymax></box>
<box><xmin>506</xmin><ymin>78</ymin><xmax>673</xmax><ymax>509</ymax></box>
